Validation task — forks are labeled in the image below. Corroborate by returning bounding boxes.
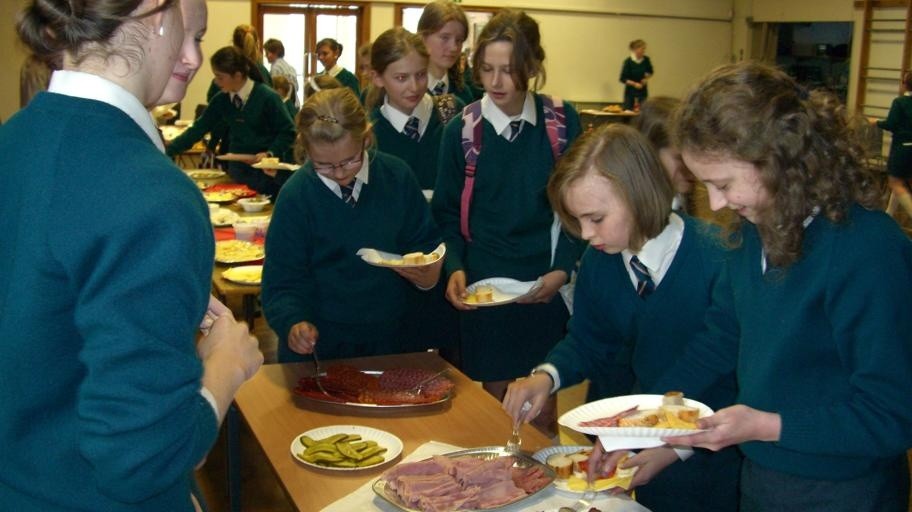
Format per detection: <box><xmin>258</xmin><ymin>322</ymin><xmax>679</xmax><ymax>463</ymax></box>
<box><xmin>503</xmin><ymin>401</ymin><xmax>533</xmax><ymax>451</ymax></box>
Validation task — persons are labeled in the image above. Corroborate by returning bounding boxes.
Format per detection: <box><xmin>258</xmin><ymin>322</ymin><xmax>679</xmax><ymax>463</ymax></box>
<box><xmin>803</xmin><ymin>39</ymin><xmax>849</xmax><ymax>90</ymax></box>
<box><xmin>582</xmin><ymin>57</ymin><xmax>910</xmax><ymax>512</ymax></box>
<box><xmin>165</xmin><ymin>3</ymin><xmax>584</xmax><ymax>443</ymax></box>
<box><xmin>629</xmin><ymin>93</ymin><xmax>698</xmax><ymax>215</ymax></box>
<box><xmin>867</xmin><ymin>71</ymin><xmax>911</xmax><ymax>194</ymax></box>
<box><xmin>2</xmin><ymin>0</ymin><xmax>266</xmax><ymax>507</ymax></box>
<box><xmin>617</xmin><ymin>38</ymin><xmax>654</xmax><ymax>108</ymax></box>
<box><xmin>502</xmin><ymin>121</ymin><xmax>736</xmax><ymax>511</ymax></box>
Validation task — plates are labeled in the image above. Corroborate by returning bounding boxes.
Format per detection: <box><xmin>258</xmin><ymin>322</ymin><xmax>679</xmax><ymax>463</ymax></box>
<box><xmin>532</xmin><ymin>444</ymin><xmax>636</xmax><ymax>494</ymax></box>
<box><xmin>189</xmin><ymin>168</ymin><xmax>271</xmax><ymax>240</ymax></box>
<box><xmin>222</xmin><ymin>265</ymin><xmax>261</xmax><ymax>285</ymax></box>
<box><xmin>215</xmin><ymin>153</ymin><xmax>256</xmax><ymax>160</ymax></box>
<box><xmin>373</xmin><ymin>445</ymin><xmax>555</xmax><ymax>511</ymax></box>
<box><xmin>214</xmin><ymin>240</ymin><xmax>265</xmax><ymax>263</ymax></box>
<box><xmin>358</xmin><ymin>242</ymin><xmax>546</xmax><ymax>307</ymax></box>
<box><xmin>298</xmin><ymin>370</ymin><xmax>453</xmax><ymax>409</ymax></box>
<box><xmin>557</xmin><ymin>393</ymin><xmax>716</xmax><ymax>436</ymax></box>
<box><xmin>250</xmin><ymin>162</ymin><xmax>298</xmax><ymax>169</ymax></box>
<box><xmin>288</xmin><ymin>423</ymin><xmax>403</xmax><ymax>472</ymax></box>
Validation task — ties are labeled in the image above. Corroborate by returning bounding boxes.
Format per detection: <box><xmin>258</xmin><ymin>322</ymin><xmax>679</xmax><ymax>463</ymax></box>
<box><xmin>340</xmin><ymin>177</ymin><xmax>356</xmax><ymax>209</ymax></box>
<box><xmin>630</xmin><ymin>255</ymin><xmax>655</xmax><ymax>299</ymax></box>
<box><xmin>434</xmin><ymin>81</ymin><xmax>445</xmax><ymax>95</ymax></box>
<box><xmin>404</xmin><ymin>116</ymin><xmax>420</xmax><ymax>142</ymax></box>
<box><xmin>510</xmin><ymin>121</ymin><xmax>521</xmax><ymax>140</ymax></box>
<box><xmin>233</xmin><ymin>94</ymin><xmax>242</xmax><ymax>110</ymax></box>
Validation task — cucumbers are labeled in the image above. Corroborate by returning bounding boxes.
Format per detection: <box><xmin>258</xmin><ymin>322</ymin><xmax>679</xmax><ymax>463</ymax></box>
<box><xmin>296</xmin><ymin>433</ymin><xmax>388</xmax><ymax>468</ymax></box>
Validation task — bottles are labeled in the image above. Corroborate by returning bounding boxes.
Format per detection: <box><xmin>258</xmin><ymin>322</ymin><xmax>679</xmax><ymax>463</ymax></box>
<box><xmin>633</xmin><ymin>97</ymin><xmax>642</xmax><ymax>113</ymax></box>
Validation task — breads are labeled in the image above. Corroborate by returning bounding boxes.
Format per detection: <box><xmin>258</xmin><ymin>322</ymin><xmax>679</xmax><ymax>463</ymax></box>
<box><xmin>402</xmin><ymin>251</ymin><xmax>425</xmax><ymax>267</ymax></box>
<box><xmin>567</xmin><ymin>453</ymin><xmax>589</xmax><ymax>473</ymax></box>
<box><xmin>617</xmin><ymin>410</ymin><xmax>658</xmax><ymax>430</ymax></box>
<box><xmin>544</xmin><ymin>452</ymin><xmax>573</xmax><ymax>479</ymax></box>
<box><xmin>475</xmin><ymin>291</ymin><xmax>493</xmax><ymax>302</ymax></box>
<box><xmin>658</xmin><ymin>404</ymin><xmax>700</xmax><ymax>423</ymax></box>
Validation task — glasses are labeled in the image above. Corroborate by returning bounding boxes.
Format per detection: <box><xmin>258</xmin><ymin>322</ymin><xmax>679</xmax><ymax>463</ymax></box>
<box><xmin>314</xmin><ymin>138</ymin><xmax>364</xmax><ymax>174</ymax></box>
